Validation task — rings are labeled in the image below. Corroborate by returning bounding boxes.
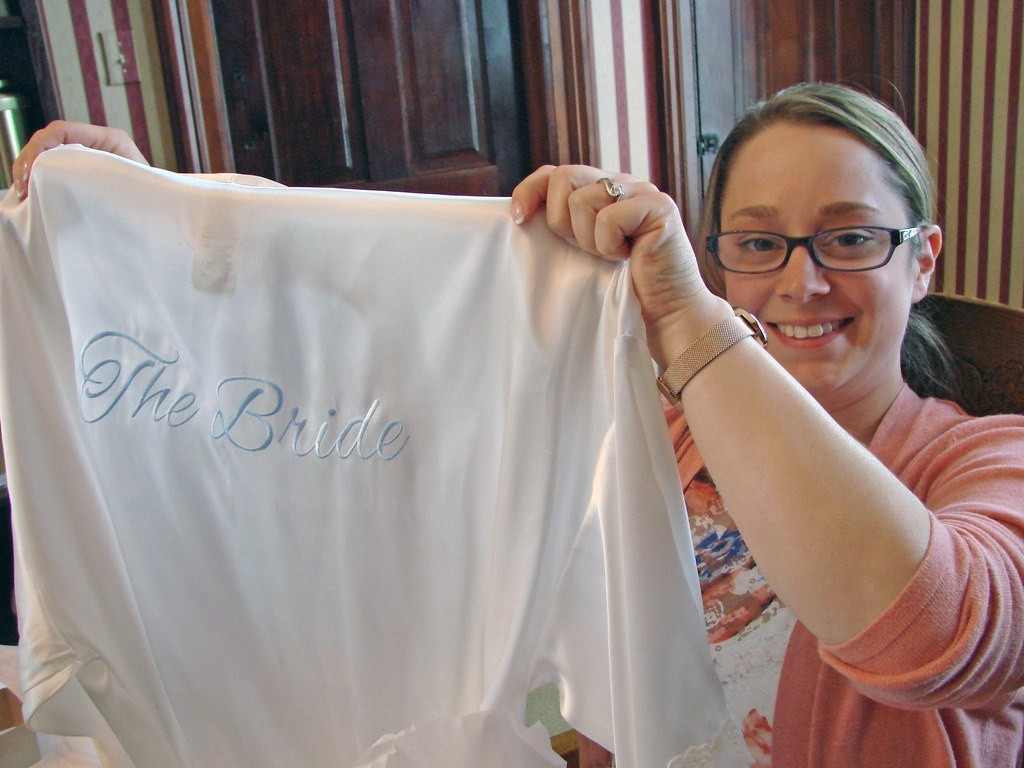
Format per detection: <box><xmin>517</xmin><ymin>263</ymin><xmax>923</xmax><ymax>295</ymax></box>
<box><xmin>596</xmin><ymin>177</ymin><xmax>624</xmax><ymax>203</ymax></box>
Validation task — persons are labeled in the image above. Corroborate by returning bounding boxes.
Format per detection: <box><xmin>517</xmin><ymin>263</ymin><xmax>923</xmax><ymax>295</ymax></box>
<box><xmin>12</xmin><ymin>84</ymin><xmax>1024</xmax><ymax>768</ymax></box>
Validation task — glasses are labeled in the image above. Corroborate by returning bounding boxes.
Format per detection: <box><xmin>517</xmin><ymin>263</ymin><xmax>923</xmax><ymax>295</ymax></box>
<box><xmin>705</xmin><ymin>226</ymin><xmax>923</xmax><ymax>274</ymax></box>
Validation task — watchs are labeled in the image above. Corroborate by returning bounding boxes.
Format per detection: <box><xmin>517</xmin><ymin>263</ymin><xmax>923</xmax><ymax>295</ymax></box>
<box><xmin>656</xmin><ymin>308</ymin><xmax>769</xmax><ymax>406</ymax></box>
